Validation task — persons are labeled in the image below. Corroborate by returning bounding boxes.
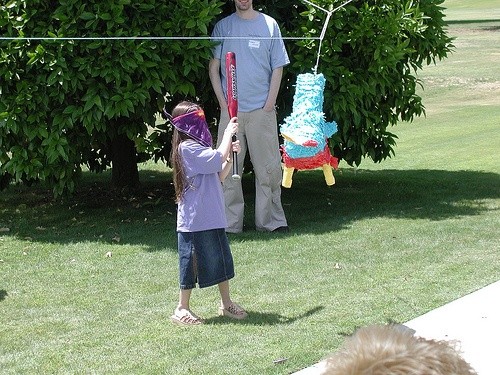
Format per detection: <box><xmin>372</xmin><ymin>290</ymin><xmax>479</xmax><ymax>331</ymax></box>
<box><xmin>208</xmin><ymin>0</ymin><xmax>291</xmax><ymax>235</ymax></box>
<box><xmin>320</xmin><ymin>323</ymin><xmax>477</xmax><ymax>375</ymax></box>
<box><xmin>169</xmin><ymin>101</ymin><xmax>249</xmax><ymax>326</ymax></box>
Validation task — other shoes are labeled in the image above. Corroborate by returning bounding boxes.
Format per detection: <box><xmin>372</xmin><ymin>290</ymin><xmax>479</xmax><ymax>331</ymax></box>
<box><xmin>275</xmin><ymin>226</ymin><xmax>290</xmax><ymax>234</ymax></box>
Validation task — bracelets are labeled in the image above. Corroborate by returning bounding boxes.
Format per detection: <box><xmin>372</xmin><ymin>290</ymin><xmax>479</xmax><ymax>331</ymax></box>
<box><xmin>226</xmin><ymin>159</ymin><xmax>233</xmax><ymax>164</ymax></box>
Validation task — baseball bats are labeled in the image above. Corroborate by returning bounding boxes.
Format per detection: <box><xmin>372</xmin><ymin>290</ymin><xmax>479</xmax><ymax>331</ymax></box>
<box><xmin>226</xmin><ymin>51</ymin><xmax>241</xmax><ymax>180</ymax></box>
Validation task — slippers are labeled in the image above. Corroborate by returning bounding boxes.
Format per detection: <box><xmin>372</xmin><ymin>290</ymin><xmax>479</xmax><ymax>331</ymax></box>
<box><xmin>219</xmin><ymin>302</ymin><xmax>248</xmax><ymax>319</ymax></box>
<box><xmin>171</xmin><ymin>309</ymin><xmax>206</xmax><ymax>328</ymax></box>
